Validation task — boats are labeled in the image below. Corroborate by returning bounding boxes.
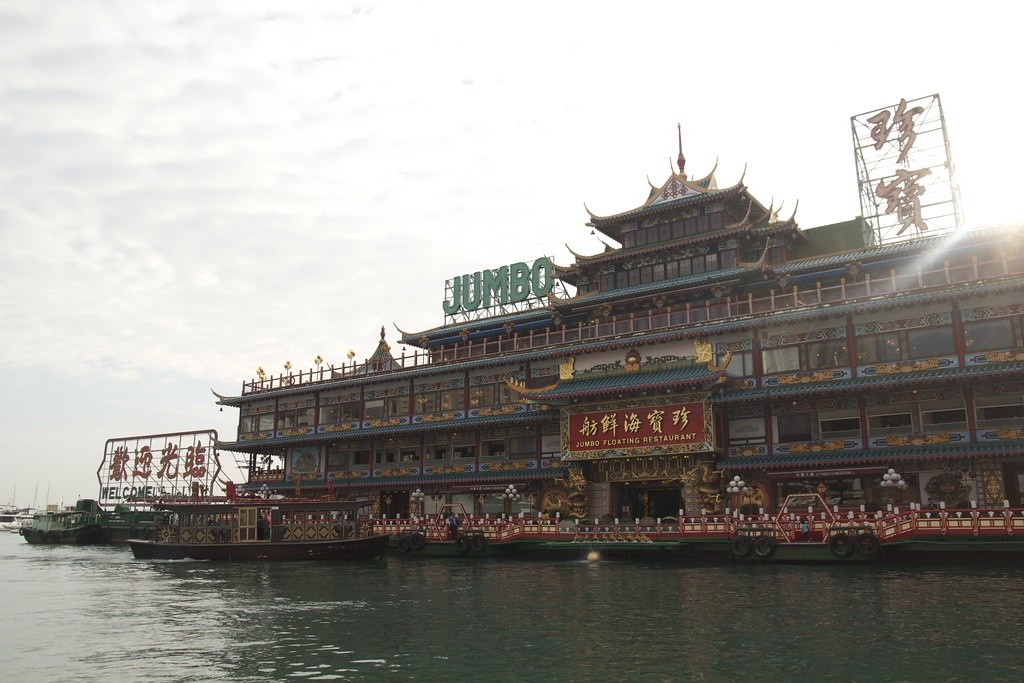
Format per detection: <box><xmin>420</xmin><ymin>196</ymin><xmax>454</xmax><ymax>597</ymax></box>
<box><xmin>0</xmin><ymin>504</ymin><xmax>110</xmax><ymax>545</ymax></box>
<box><xmin>126</xmin><ymin>481</ymin><xmax>394</xmax><ymax>561</ymax></box>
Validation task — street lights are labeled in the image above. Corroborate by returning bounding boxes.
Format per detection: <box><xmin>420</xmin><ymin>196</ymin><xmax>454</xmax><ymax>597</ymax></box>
<box><xmin>258</xmin><ymin>483</ymin><xmax>271</xmax><ymax>500</ymax></box>
<box><xmin>727</xmin><ymin>475</ymin><xmax>748</xmax><ymax>521</ymax></box>
<box><xmin>880</xmin><ymin>469</ymin><xmax>904</xmax><ymax>513</ymax></box>
<box><xmin>410</xmin><ymin>488</ymin><xmax>426</xmax><ymax>524</ymax></box>
<box><xmin>502</xmin><ymin>484</ymin><xmax>520</xmax><ymax>523</ymax></box>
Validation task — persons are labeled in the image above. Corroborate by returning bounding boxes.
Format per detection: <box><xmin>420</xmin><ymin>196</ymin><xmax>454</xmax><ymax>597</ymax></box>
<box><xmin>449</xmin><ymin>512</ymin><xmax>459</xmax><ymax>540</ymax></box>
<box><xmin>800</xmin><ymin>521</ymin><xmax>811</xmax><ymax>542</ymax></box>
<box><xmin>443</xmin><ymin>509</ymin><xmax>451</xmax><ymax>519</ymax></box>
<box><xmin>257</xmin><ymin>516</ymin><xmax>264</xmax><ymax>540</ymax></box>
<box><xmin>927</xmin><ymin>498</ymin><xmax>940</xmax><ymax>518</ymax></box>
